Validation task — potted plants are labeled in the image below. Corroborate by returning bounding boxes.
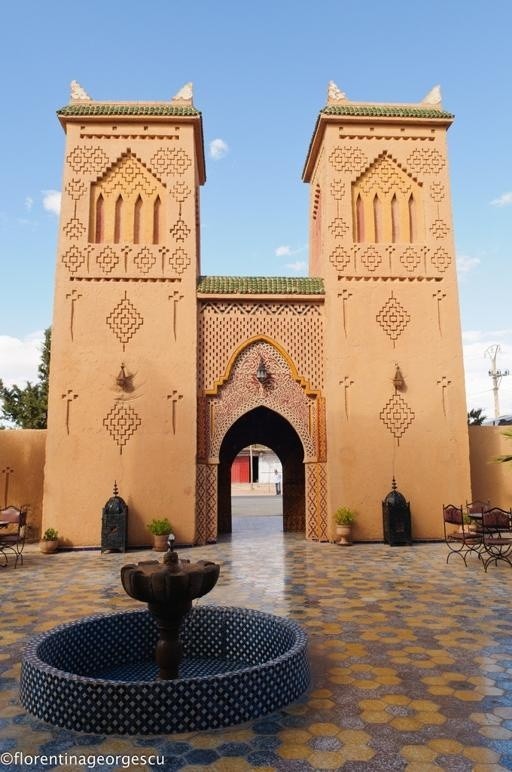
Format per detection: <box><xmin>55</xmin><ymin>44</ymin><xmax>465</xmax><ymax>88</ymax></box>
<box><xmin>39</xmin><ymin>528</ymin><xmax>58</xmax><ymax>553</ymax></box>
<box><xmin>146</xmin><ymin>519</ymin><xmax>173</xmax><ymax>551</ymax></box>
<box><xmin>333</xmin><ymin>505</ymin><xmax>354</xmax><ymax>545</ymax></box>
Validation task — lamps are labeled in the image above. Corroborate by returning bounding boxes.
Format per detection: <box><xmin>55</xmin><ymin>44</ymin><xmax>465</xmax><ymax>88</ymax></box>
<box><xmin>393</xmin><ymin>366</ymin><xmax>404</xmax><ymax>389</ymax></box>
<box><xmin>256</xmin><ymin>360</ymin><xmax>267</xmax><ymax>382</ymax></box>
<box><xmin>116</xmin><ymin>366</ymin><xmax>126</xmax><ymax>385</ymax></box>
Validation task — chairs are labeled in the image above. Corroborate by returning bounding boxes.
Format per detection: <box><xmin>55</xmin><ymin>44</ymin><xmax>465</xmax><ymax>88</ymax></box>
<box><xmin>443</xmin><ymin>499</ymin><xmax>512</xmax><ymax>572</ymax></box>
<box><xmin>0</xmin><ymin>504</ymin><xmax>29</xmax><ymax>569</ymax></box>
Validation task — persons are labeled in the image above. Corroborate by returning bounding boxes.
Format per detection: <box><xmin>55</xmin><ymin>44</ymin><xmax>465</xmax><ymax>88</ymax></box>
<box><xmin>273</xmin><ymin>469</ymin><xmax>281</xmax><ymax>496</ymax></box>
